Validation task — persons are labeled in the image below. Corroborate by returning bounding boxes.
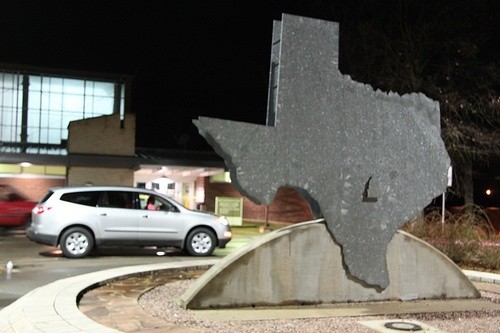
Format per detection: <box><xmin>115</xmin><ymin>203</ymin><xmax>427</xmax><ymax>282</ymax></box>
<box><xmin>143</xmin><ymin>195</ymin><xmax>161</xmax><ymax>211</ymax></box>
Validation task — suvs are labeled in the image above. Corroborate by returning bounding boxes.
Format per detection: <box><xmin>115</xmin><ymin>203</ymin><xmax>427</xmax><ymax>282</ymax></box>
<box><xmin>25</xmin><ymin>186</ymin><xmax>232</xmax><ymax>257</ymax></box>
